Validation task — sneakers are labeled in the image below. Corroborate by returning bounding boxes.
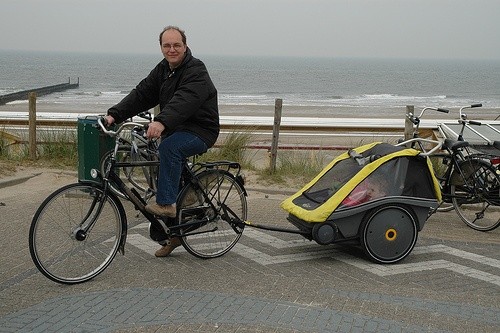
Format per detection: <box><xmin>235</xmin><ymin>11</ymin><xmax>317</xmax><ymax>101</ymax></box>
<box><xmin>145</xmin><ymin>202</ymin><xmax>176</xmax><ymax>218</ymax></box>
<box><xmin>155</xmin><ymin>234</ymin><xmax>185</xmax><ymax>257</ymax></box>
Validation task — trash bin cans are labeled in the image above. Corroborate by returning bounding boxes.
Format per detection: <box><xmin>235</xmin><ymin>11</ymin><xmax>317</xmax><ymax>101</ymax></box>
<box><xmin>77</xmin><ymin>114</ymin><xmax>121</xmax><ymax>193</ymax></box>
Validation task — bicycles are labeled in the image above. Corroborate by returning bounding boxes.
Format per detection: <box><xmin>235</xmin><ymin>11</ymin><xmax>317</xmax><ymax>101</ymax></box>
<box><xmin>97</xmin><ymin>109</ymin><xmax>209</xmax><ymax>217</ymax></box>
<box><xmin>396</xmin><ymin>103</ymin><xmax>500</xmax><ymax>232</ymax></box>
<box><xmin>27</xmin><ymin>109</ymin><xmax>249</xmax><ymax>284</ymax></box>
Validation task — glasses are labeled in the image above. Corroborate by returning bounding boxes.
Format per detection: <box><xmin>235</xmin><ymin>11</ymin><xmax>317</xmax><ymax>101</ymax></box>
<box><xmin>160</xmin><ymin>43</ymin><xmax>185</xmax><ymax>49</ymax></box>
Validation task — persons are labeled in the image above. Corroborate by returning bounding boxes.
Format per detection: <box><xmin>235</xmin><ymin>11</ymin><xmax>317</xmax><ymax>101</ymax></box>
<box><xmin>367</xmin><ymin>176</ymin><xmax>388</xmax><ymax>201</ymax></box>
<box><xmin>100</xmin><ymin>26</ymin><xmax>220</xmax><ymax>257</ymax></box>
